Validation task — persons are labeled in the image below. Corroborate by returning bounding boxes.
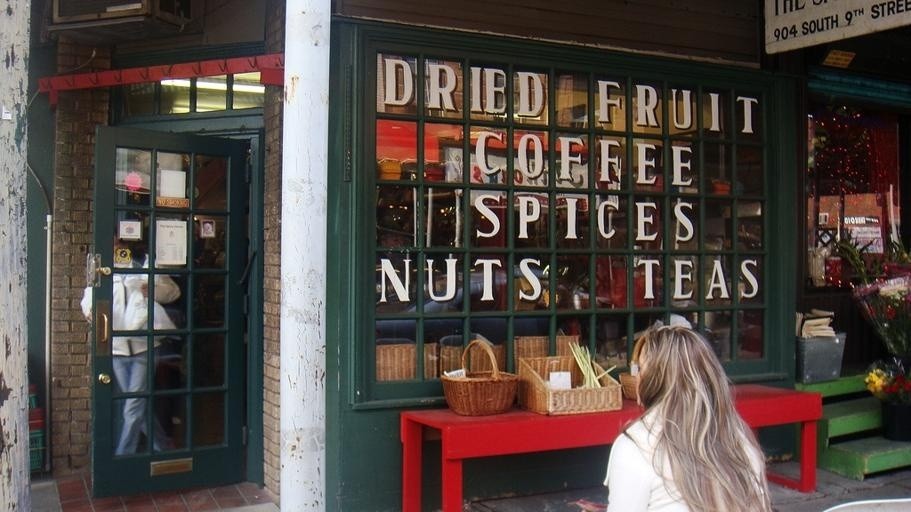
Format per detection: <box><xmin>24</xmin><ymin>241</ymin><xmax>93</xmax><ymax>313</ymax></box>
<box><xmin>80</xmin><ymin>228</ymin><xmax>181</xmax><ymax>455</ymax></box>
<box><xmin>582</xmin><ymin>327</ymin><xmax>772</xmax><ymax>512</ymax></box>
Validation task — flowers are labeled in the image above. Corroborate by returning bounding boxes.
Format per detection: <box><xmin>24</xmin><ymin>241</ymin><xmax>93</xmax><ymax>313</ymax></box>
<box><xmin>863</xmin><ymin>364</ymin><xmax>911</xmax><ymax>404</ymax></box>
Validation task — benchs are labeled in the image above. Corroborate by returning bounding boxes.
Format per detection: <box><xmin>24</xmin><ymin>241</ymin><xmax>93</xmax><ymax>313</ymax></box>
<box><xmin>392</xmin><ymin>387</ymin><xmax>824</xmax><ymax>512</ymax></box>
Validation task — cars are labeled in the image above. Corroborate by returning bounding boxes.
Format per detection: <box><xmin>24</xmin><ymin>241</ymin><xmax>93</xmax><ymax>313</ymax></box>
<box><xmin>376</xmin><ymin>267</ymin><xmax>639</xmax><ymax>343</ymax></box>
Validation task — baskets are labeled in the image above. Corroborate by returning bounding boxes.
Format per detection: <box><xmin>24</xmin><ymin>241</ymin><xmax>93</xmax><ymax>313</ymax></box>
<box><xmin>440</xmin><ymin>335</ymin><xmax>637</xmax><ymax>416</ymax></box>
<box><xmin>375</xmin><ymin>342</ymin><xmax>436</xmax><ymax>381</ymax></box>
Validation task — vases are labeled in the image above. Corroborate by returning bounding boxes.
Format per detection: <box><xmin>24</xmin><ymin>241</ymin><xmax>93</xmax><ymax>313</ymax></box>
<box><xmin>879</xmin><ymin>396</ymin><xmax>910</xmax><ymax>443</ymax></box>
